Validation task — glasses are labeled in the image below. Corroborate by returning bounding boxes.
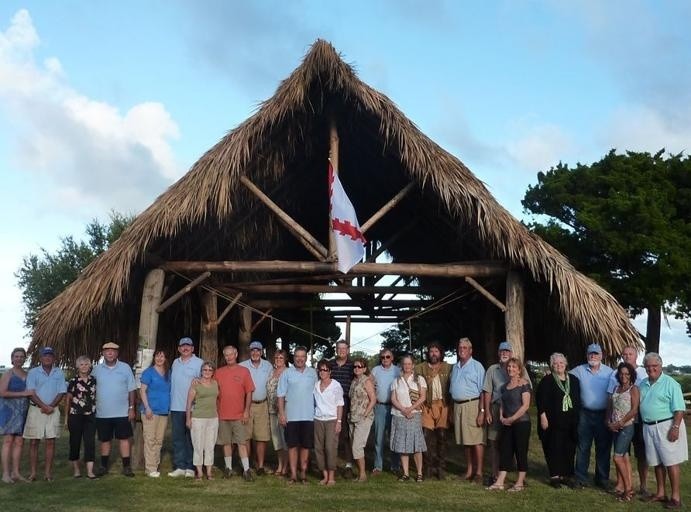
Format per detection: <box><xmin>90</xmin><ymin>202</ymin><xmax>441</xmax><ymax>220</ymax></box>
<box><xmin>318</xmin><ymin>354</ymin><xmax>390</xmax><ymax>372</ymax></box>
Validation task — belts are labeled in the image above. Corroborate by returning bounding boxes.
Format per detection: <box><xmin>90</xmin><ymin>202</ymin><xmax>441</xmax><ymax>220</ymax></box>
<box><xmin>644</xmin><ymin>416</ymin><xmax>673</xmax><ymax>427</ymax></box>
<box><xmin>251</xmin><ymin>397</ymin><xmax>267</xmax><ymax>405</ymax></box>
<box><xmin>374</xmin><ymin>400</ymin><xmax>391</xmax><ymax>406</ymax></box>
<box><xmin>454</xmin><ymin>396</ymin><xmax>479</xmax><ymax>405</ymax></box>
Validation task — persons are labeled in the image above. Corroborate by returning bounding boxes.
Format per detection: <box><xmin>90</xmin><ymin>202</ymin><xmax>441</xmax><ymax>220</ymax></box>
<box><xmin>0</xmin><ymin>342</ymin><xmax>137</xmax><ymax>485</ymax></box>
<box><xmin>535</xmin><ymin>342</ymin><xmax>690</xmax><ymax>510</ymax></box>
<box><xmin>139</xmin><ymin>338</ymin><xmax>533</xmax><ymax>492</ymax></box>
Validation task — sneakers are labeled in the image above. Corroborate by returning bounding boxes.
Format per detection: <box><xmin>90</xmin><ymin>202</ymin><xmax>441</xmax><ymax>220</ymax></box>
<box><xmin>256</xmin><ymin>467</ymin><xmax>446</xmax><ymax>485</ymax></box>
<box><xmin>122</xmin><ymin>465</ymin><xmax>135</xmax><ymax>477</ymax></box>
<box><xmin>550</xmin><ymin>474</ymin><xmax>681</xmax><ymax>509</ymax></box>
<box><xmin>167</xmin><ymin>467</ymin><xmax>184</xmax><ymax>479</ymax></box>
<box><xmin>242</xmin><ymin>468</ymin><xmax>253</xmax><ymax>482</ymax></box>
<box><xmin>185</xmin><ymin>469</ymin><xmax>214</xmax><ymax>481</ymax></box>
<box><xmin>96</xmin><ymin>467</ymin><xmax>109</xmax><ymax>477</ymax></box>
<box><xmin>222</xmin><ymin>467</ymin><xmax>233</xmax><ymax>480</ymax></box>
<box><xmin>87</xmin><ymin>472</ymin><xmax>101</xmax><ymax>481</ymax></box>
<box><xmin>73</xmin><ymin>473</ymin><xmax>83</xmax><ymax>481</ymax></box>
<box><xmin>145</xmin><ymin>469</ymin><xmax>162</xmax><ymax>480</ymax></box>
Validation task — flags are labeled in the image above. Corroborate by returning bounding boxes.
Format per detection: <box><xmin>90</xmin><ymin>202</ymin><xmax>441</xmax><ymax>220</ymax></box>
<box><xmin>327</xmin><ymin>160</ymin><xmax>367</xmax><ymax>276</ymax></box>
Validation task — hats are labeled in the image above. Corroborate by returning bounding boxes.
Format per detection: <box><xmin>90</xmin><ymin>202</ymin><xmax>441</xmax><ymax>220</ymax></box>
<box><xmin>178</xmin><ymin>336</ymin><xmax>193</xmax><ymax>346</ymax></box>
<box><xmin>103</xmin><ymin>342</ymin><xmax>119</xmax><ymax>350</ymax></box>
<box><xmin>498</xmin><ymin>342</ymin><xmax>513</xmax><ymax>353</ymax></box>
<box><xmin>249</xmin><ymin>341</ymin><xmax>263</xmax><ymax>351</ymax></box>
<box><xmin>39</xmin><ymin>346</ymin><xmax>55</xmax><ymax>357</ymax></box>
<box><xmin>586</xmin><ymin>343</ymin><xmax>602</xmax><ymax>355</ymax></box>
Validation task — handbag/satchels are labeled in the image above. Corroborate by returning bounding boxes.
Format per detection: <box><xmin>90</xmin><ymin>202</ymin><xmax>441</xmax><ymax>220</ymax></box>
<box><xmin>403</xmin><ymin>376</ymin><xmax>424</xmax><ymax>409</ymax></box>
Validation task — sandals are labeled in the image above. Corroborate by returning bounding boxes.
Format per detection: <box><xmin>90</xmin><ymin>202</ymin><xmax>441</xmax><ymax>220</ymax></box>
<box><xmin>1</xmin><ymin>471</ymin><xmax>54</xmax><ymax>484</ymax></box>
<box><xmin>460</xmin><ymin>466</ymin><xmax>525</xmax><ymax>494</ymax></box>
